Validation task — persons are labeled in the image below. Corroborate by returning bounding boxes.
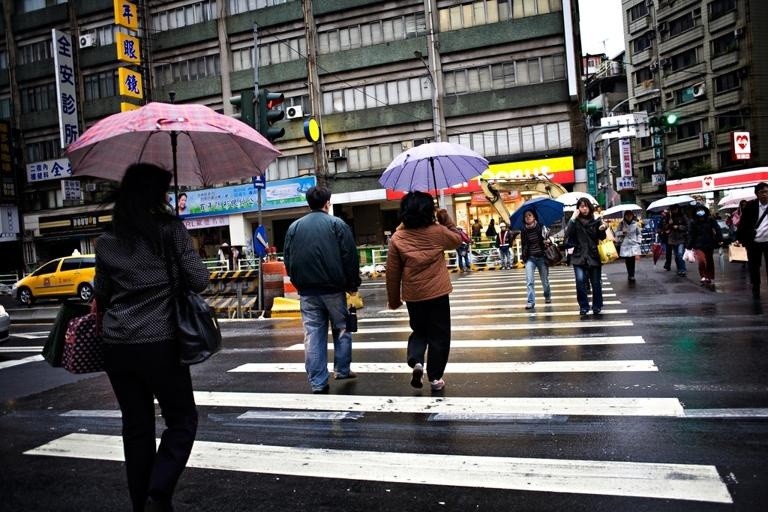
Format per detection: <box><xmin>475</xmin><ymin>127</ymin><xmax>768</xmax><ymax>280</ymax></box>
<box><xmin>521</xmin><ymin>207</ymin><xmax>554</xmax><ymax>309</ymax></box>
<box><xmin>486</xmin><ymin>218</ymin><xmax>497</xmax><ymax>248</ymax></box>
<box><xmin>456</xmin><ymin>226</ymin><xmax>474</xmax><ymax>273</ymax></box>
<box><xmin>563</xmin><ymin>217</ymin><xmax>574</xmax><ymax>266</ymax></box>
<box><xmin>283</xmin><ymin>186</ymin><xmax>362</xmax><ymax>394</ymax></box>
<box><xmin>564</xmin><ymin>197</ymin><xmax>608</xmax><ymax>315</ymax></box>
<box><xmin>472</xmin><ymin>219</ymin><xmax>483</xmax><ymax>248</ymax></box>
<box><xmin>386</xmin><ymin>190</ymin><xmax>462</xmax><ymax>390</ymax></box>
<box><xmin>662</xmin><ymin>202</ymin><xmax>725</xmax><ymax>283</ymax></box>
<box><xmin>174</xmin><ymin>192</ymin><xmax>189</xmax><ymax>214</ymax></box>
<box><xmin>615</xmin><ymin>210</ymin><xmax>642</xmax><ymax>288</ymax></box>
<box><xmin>726</xmin><ymin>182</ymin><xmax>767</xmax><ymax>314</ymax></box>
<box><xmin>633</xmin><ymin>212</ymin><xmax>666</xmax><ymax>252</ymax></box>
<box><xmin>91</xmin><ymin>163</ymin><xmax>207</xmax><ymax>511</ymax></box>
<box><xmin>494</xmin><ymin>220</ymin><xmax>513</xmax><ymax>270</ymax></box>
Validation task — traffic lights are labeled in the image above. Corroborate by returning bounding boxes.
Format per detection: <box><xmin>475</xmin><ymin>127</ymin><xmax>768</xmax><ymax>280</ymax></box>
<box><xmin>258</xmin><ymin>88</ymin><xmax>285</xmax><ymax>142</ymax></box>
<box><xmin>227</xmin><ymin>91</ymin><xmax>254</xmax><ymax>127</ymax></box>
<box><xmin>646</xmin><ymin>113</ymin><xmax>677</xmax><ymax>126</ymax></box>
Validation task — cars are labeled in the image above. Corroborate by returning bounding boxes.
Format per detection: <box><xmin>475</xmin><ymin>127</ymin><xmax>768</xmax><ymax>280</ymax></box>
<box><xmin>0</xmin><ymin>304</ymin><xmax>11</xmax><ymax>343</ymax></box>
<box><xmin>0</xmin><ymin>283</ymin><xmax>12</xmax><ymax>308</ymax></box>
<box><xmin>10</xmin><ymin>249</ymin><xmax>97</xmax><ymax>303</ymax></box>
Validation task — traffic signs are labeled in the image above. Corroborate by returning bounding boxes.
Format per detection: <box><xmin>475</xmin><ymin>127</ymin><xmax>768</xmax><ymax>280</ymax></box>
<box><xmin>253</xmin><ymin>171</ymin><xmax>267</xmax><ymax>188</ymax></box>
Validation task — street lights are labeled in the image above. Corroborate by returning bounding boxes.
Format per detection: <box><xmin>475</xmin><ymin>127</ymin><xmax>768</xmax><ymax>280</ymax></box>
<box><xmin>604</xmin><ymin>136</ymin><xmax>636</xmax><ymax>208</ymax></box>
<box><xmin>602</xmin><ymin>88</ymin><xmax>660</xmax><ymax>210</ymax></box>
<box><xmin>412</xmin><ymin>49</ymin><xmax>438</xmax><ymax>143</ymax></box>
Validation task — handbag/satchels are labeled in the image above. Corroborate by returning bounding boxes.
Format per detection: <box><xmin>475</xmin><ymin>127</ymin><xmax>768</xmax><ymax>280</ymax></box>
<box><xmin>727</xmin><ymin>243</ymin><xmax>749</xmax><ymax>263</ymax></box>
<box><xmin>544</xmin><ymin>244</ymin><xmax>562</xmax><ymax>266</ymax></box>
<box><xmin>169</xmin><ymin>291</ymin><xmax>222</xmax><ymax>364</ymax></box>
<box><xmin>597</xmin><ymin>240</ymin><xmax>619</xmax><ymax>263</ymax></box>
<box><xmin>41</xmin><ymin>301</ymin><xmax>102</xmax><ymax>374</ymax></box>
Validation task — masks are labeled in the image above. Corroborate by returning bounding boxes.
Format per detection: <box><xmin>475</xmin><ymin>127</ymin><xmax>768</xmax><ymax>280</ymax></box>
<box><xmin>697</xmin><ymin>211</ymin><xmax>705</xmax><ymax>216</ymax></box>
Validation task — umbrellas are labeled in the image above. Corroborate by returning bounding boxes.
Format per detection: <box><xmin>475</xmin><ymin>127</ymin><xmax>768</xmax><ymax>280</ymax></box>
<box><xmin>378</xmin><ymin>141</ymin><xmax>490</xmax><ymax>206</ymax></box>
<box><xmin>63</xmin><ymin>102</ymin><xmax>281</xmax><ymax>217</ymax></box>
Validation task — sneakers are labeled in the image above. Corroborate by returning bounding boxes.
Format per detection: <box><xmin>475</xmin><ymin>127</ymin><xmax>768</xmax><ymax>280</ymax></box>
<box><xmin>410</xmin><ymin>364</ymin><xmax>424</xmax><ymax>388</ymax></box>
<box><xmin>432</xmin><ymin>378</ymin><xmax>445</xmax><ymax>391</ymax></box>
<box><xmin>334</xmin><ymin>371</ymin><xmax>356</xmax><ymax>379</ymax></box>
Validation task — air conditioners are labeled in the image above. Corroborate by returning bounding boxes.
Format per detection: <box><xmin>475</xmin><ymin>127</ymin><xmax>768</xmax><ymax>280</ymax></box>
<box><xmin>693</xmin><ymin>84</ymin><xmax>705</xmax><ymax>97</ymax></box>
<box><xmin>86</xmin><ymin>184</ymin><xmax>96</xmax><ymax>191</ymax></box>
<box><xmin>79</xmin><ymin>34</ymin><xmax>92</xmax><ymax>48</ymax></box>
<box><xmin>287</xmin><ymin>106</ymin><xmax>303</xmax><ymax>118</ymax></box>
